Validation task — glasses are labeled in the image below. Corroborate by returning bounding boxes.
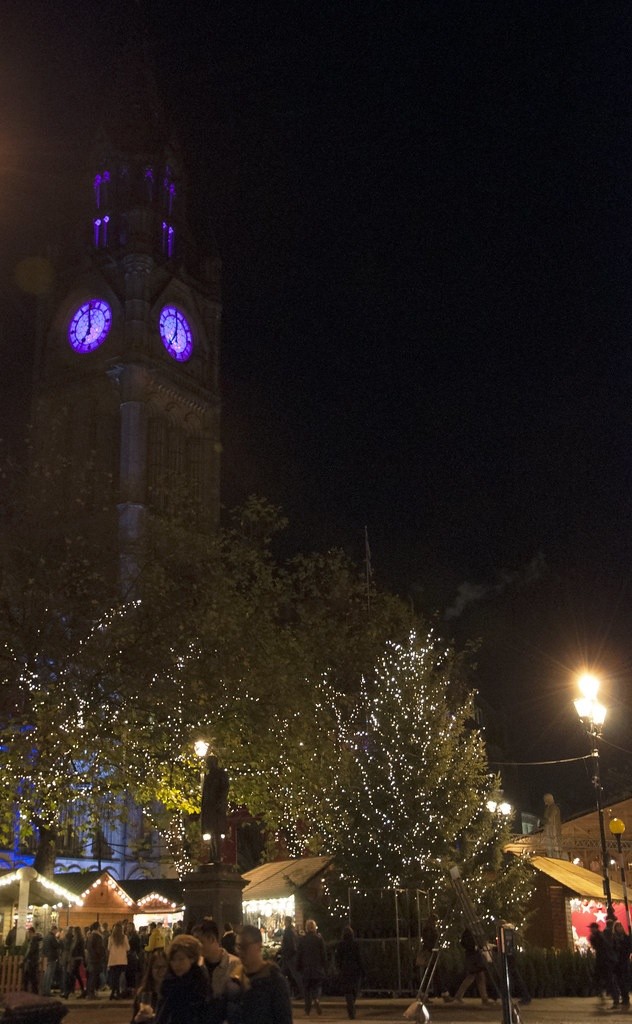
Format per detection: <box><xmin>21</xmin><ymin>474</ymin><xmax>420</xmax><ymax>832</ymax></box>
<box><xmin>232</xmin><ymin>942</ymin><xmax>251</xmax><ymax>953</ymax></box>
<box><xmin>151</xmin><ymin>965</ymin><xmax>167</xmax><ymax>970</ymax></box>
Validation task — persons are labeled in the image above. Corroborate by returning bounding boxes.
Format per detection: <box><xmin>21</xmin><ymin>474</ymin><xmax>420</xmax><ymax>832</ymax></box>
<box><xmin>421</xmin><ymin>915</ymin><xmax>532</xmax><ymax>1004</ymax></box>
<box><xmin>0</xmin><ymin>916</ymin><xmax>363</xmax><ymax>1024</ymax></box>
<box><xmin>201</xmin><ymin>756</ymin><xmax>230</xmax><ymax>864</ymax></box>
<box><xmin>587</xmin><ymin>921</ymin><xmax>632</xmax><ymax>1012</ymax></box>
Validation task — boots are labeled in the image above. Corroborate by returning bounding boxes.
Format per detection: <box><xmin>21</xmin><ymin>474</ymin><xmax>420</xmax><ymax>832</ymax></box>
<box><xmin>455</xmin><ymin>985</ymin><xmax>466</xmax><ymax>1005</ymax></box>
<box><xmin>480</xmin><ymin>995</ymin><xmax>494</xmax><ymax>1006</ymax></box>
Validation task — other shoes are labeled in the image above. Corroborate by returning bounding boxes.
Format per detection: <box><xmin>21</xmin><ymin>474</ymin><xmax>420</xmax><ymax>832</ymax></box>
<box><xmin>315</xmin><ymin>1002</ymin><xmax>323</xmax><ymax>1016</ymax></box>
<box><xmin>109</xmin><ymin>995</ymin><xmax>119</xmax><ymax>1000</ymax></box>
<box><xmin>59</xmin><ymin>994</ymin><xmax>68</xmax><ymax>999</ymax></box>
<box><xmin>77</xmin><ymin>991</ymin><xmax>87</xmax><ymax>998</ymax></box>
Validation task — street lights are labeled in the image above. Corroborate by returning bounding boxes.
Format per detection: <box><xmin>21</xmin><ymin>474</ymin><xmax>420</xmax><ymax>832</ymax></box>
<box><xmin>610</xmin><ymin>817</ymin><xmax>632</xmax><ymax>933</ymax></box>
<box><xmin>571</xmin><ymin>668</ymin><xmax>618</xmax><ymax>922</ymax></box>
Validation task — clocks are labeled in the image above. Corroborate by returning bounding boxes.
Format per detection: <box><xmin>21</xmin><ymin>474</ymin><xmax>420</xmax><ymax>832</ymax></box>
<box><xmin>158</xmin><ymin>301</ymin><xmax>195</xmax><ymax>362</ymax></box>
<box><xmin>66</xmin><ymin>298</ymin><xmax>113</xmax><ymax>354</ymax></box>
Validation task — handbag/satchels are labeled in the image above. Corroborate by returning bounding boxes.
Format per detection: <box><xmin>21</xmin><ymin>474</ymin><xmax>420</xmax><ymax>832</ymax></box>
<box><xmin>97</xmin><ymin>971</ymin><xmax>106</xmax><ymax>990</ymax></box>
<box><xmin>416</xmin><ymin>941</ymin><xmax>429</xmax><ymax>966</ymax></box>
<box><xmin>53</xmin><ymin>958</ymin><xmax>63</xmax><ymax>981</ymax></box>
<box><xmin>66</xmin><ymin>959</ymin><xmax>76</xmax><ymax>974</ymax></box>
<box><xmin>134</xmin><ymin>1003</ymin><xmax>157</xmax><ymax>1022</ymax></box>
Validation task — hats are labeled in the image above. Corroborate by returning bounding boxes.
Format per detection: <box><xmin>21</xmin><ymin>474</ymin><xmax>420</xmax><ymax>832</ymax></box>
<box><xmin>148</xmin><ymin>922</ymin><xmax>156</xmax><ymax>927</ymax></box>
<box><xmin>587</xmin><ymin>922</ymin><xmax>599</xmax><ymax>928</ymax></box>
<box><xmin>304</xmin><ymin>919</ymin><xmax>318</xmax><ymax>929</ymax></box>
